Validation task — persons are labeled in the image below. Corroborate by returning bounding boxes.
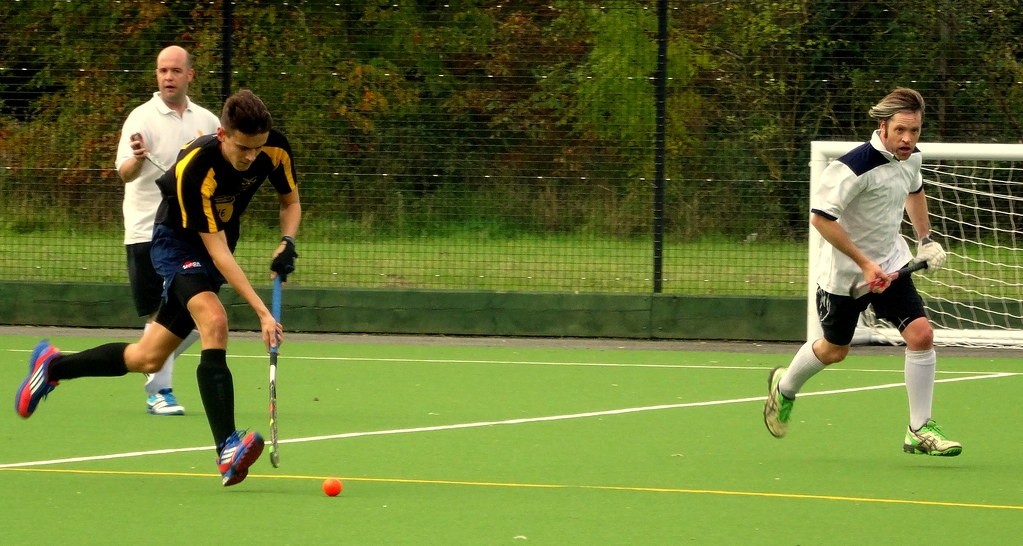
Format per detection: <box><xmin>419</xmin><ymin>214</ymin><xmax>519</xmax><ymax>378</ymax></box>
<box><xmin>115</xmin><ymin>45</ymin><xmax>221</xmax><ymax>415</ymax></box>
<box><xmin>764</xmin><ymin>85</ymin><xmax>963</xmax><ymax>457</ymax></box>
<box><xmin>16</xmin><ymin>88</ymin><xmax>300</xmax><ymax>486</ymax></box>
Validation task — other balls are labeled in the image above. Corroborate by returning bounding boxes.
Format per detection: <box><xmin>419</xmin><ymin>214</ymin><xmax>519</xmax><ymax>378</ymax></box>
<box><xmin>323</xmin><ymin>479</ymin><xmax>342</xmax><ymax>497</ymax></box>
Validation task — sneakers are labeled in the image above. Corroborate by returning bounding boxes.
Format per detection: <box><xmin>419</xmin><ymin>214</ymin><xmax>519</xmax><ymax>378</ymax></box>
<box><xmin>16</xmin><ymin>338</ymin><xmax>61</xmax><ymax>420</ymax></box>
<box><xmin>763</xmin><ymin>366</ymin><xmax>797</xmax><ymax>437</ymax></box>
<box><xmin>903</xmin><ymin>420</ymin><xmax>963</xmax><ymax>456</ymax></box>
<box><xmin>217</xmin><ymin>427</ymin><xmax>266</xmax><ymax>486</ymax></box>
<box><xmin>147</xmin><ymin>386</ymin><xmax>185</xmax><ymax>415</ymax></box>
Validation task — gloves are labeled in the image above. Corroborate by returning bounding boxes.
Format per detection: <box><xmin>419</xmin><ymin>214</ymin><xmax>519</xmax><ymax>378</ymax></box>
<box><xmin>914</xmin><ymin>234</ymin><xmax>947</xmax><ymax>276</ymax></box>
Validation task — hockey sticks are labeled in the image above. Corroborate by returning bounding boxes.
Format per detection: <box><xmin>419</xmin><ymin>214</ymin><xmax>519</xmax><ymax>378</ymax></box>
<box><xmin>269</xmin><ymin>273</ymin><xmax>281</xmax><ymax>468</ymax></box>
<box><xmin>143</xmin><ymin>152</ymin><xmax>169</xmax><ymax>172</ymax></box>
<box><xmin>849</xmin><ymin>260</ymin><xmax>927</xmax><ymax>300</ymax></box>
